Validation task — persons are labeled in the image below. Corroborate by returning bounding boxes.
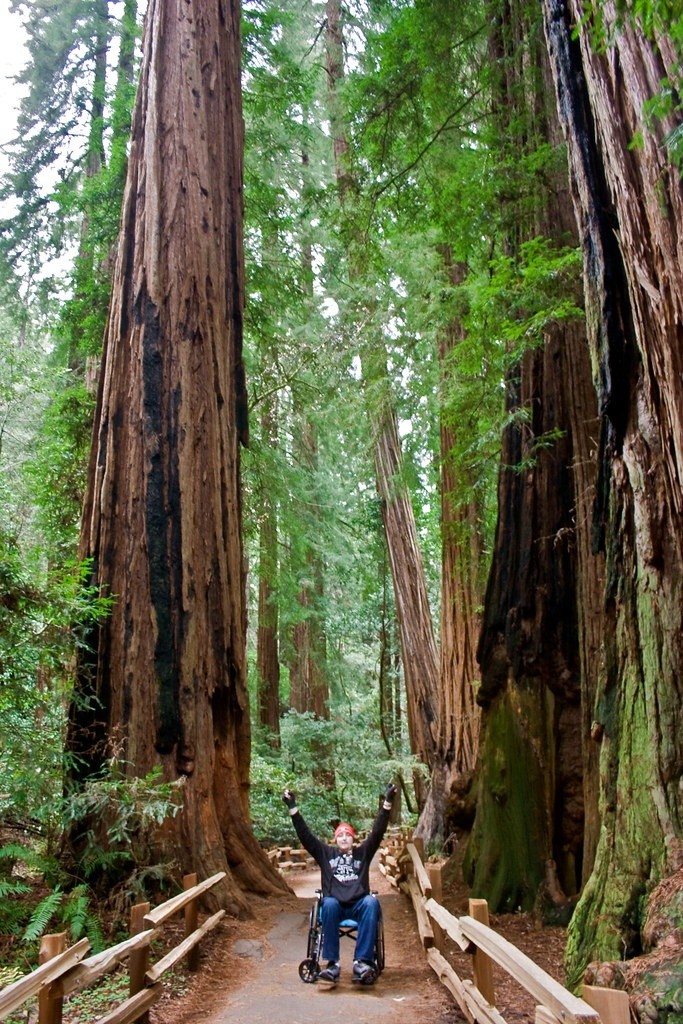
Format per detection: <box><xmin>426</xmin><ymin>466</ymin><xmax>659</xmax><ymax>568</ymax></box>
<box><xmin>282</xmin><ymin>783</ymin><xmax>397</xmax><ymax>982</ymax></box>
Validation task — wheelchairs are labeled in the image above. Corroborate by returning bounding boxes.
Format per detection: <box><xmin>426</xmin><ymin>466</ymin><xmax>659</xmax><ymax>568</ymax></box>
<box><xmin>298</xmin><ymin>889</ymin><xmax>384</xmax><ymax>986</ymax></box>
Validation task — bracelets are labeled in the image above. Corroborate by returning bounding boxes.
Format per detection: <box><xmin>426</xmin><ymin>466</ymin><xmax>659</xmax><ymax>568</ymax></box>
<box><xmin>383</xmin><ymin>804</ymin><xmax>392</xmax><ymax>807</ymax></box>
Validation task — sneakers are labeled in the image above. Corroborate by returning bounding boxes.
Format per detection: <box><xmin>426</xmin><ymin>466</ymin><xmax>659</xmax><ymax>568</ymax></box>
<box><xmin>319</xmin><ymin>963</ymin><xmax>341</xmax><ymax>983</ymax></box>
<box><xmin>352</xmin><ymin>963</ymin><xmax>374</xmax><ymax>982</ymax></box>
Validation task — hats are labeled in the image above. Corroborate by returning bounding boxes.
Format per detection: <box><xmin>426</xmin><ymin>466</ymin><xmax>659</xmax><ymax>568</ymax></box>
<box><xmin>334</xmin><ymin>822</ymin><xmax>354</xmax><ymax>840</ymax></box>
